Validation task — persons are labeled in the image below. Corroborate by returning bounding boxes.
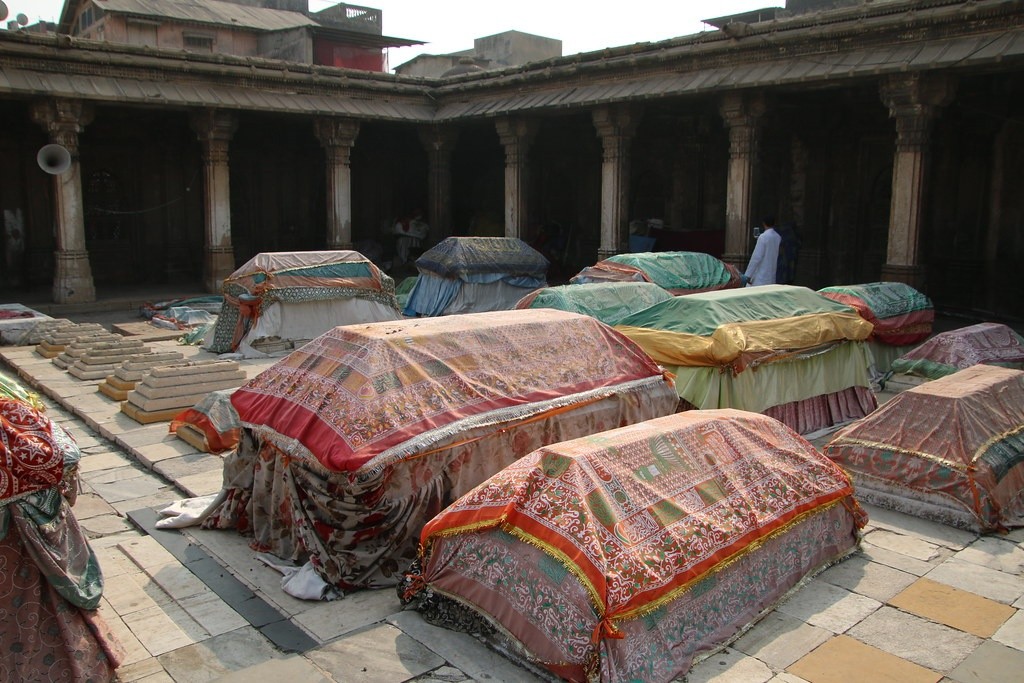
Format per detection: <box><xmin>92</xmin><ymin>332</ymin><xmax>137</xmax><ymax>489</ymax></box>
<box><xmin>743</xmin><ymin>215</ymin><xmax>781</xmax><ymax>286</ymax></box>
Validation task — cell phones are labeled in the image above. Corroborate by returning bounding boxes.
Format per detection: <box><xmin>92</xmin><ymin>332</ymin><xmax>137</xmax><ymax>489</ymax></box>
<box><xmin>753</xmin><ymin>227</ymin><xmax>760</xmax><ymax>238</ymax></box>
